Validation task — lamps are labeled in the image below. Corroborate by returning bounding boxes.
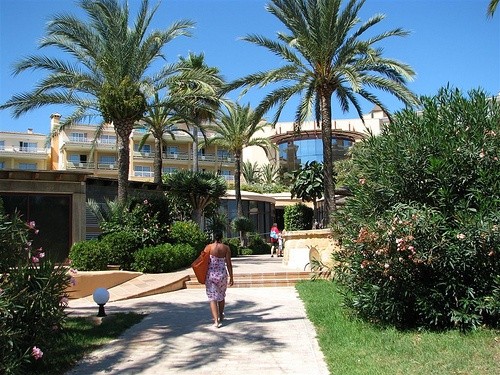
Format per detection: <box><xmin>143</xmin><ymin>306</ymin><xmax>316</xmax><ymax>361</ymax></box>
<box><xmin>93</xmin><ymin>287</ymin><xmax>110</xmax><ymax>318</ymax></box>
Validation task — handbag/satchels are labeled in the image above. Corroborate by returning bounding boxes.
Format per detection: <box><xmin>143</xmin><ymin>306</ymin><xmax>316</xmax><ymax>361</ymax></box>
<box><xmin>191</xmin><ymin>251</ymin><xmax>209</xmax><ymax>284</ymax></box>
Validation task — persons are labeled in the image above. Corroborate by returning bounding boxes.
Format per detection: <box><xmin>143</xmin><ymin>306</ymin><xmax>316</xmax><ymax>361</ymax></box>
<box><xmin>202</xmin><ymin>229</ymin><xmax>234</xmax><ymax>328</ymax></box>
<box><xmin>269</xmin><ymin>223</ymin><xmax>281</xmax><ymax>257</ymax></box>
<box><xmin>278</xmin><ymin>232</ymin><xmax>283</xmax><ymax>257</ymax></box>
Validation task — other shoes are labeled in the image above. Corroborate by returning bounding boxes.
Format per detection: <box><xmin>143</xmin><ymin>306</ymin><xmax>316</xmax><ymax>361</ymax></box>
<box><xmin>219</xmin><ymin>312</ymin><xmax>225</xmax><ymax>320</ymax></box>
<box><xmin>277</xmin><ymin>253</ymin><xmax>283</xmax><ymax>257</ymax></box>
<box><xmin>271</xmin><ymin>255</ymin><xmax>274</xmax><ymax>257</ymax></box>
<box><xmin>214</xmin><ymin>322</ymin><xmax>222</xmax><ymax>328</ymax></box>
